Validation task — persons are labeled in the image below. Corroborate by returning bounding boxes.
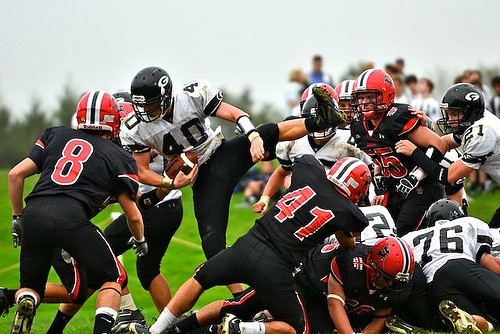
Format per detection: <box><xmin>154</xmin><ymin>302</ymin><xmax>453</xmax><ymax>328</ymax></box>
<box><xmin>8</xmin><ymin>90</ymin><xmax>148</xmax><ymax>334</ymax></box>
<box><xmin>119</xmin><ymin>67</ymin><xmax>346</xmax><ymax>299</ymax></box>
<box><xmin>160</xmin><ymin>55</ymin><xmax>500</xmax><ymax>334</ymax></box>
<box><xmin>0</xmin><ymin>92</ymin><xmax>199</xmax><ymax>334</ymax></box>
<box><xmin>128</xmin><ymin>155</ymin><xmax>371</xmax><ymax>334</ymax></box>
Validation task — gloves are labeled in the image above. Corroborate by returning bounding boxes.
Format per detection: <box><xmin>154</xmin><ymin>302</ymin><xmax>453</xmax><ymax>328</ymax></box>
<box><xmin>374</xmin><ymin>172</ymin><xmax>388</xmax><ymax>195</ymax></box>
<box><xmin>11</xmin><ymin>215</ymin><xmax>23</xmax><ymax>248</ymax></box>
<box><xmin>127</xmin><ymin>236</ymin><xmax>148</xmax><ymax>257</ymax></box>
<box><xmin>396</xmin><ymin>171</ymin><xmax>419</xmax><ymax>198</ymax></box>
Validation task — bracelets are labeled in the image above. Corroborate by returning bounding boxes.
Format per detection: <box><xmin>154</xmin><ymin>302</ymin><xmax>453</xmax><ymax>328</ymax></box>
<box><xmin>260</xmin><ymin>195</ymin><xmax>270</xmax><ymax>205</ymax></box>
<box><xmin>161</xmin><ymin>177</ymin><xmax>174</xmax><ymax>189</ymax></box>
<box><xmin>327</xmin><ymin>293</ymin><xmax>345</xmax><ymax>307</ymax></box>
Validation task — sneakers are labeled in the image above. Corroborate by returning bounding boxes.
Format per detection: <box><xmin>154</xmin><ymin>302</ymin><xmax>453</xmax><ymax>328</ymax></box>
<box><xmin>10</xmin><ymin>295</ymin><xmax>36</xmax><ymax>334</ymax></box>
<box><xmin>92</xmin><ymin>307</ymin><xmax>272</xmax><ymax>334</ymax></box>
<box><xmin>311</xmin><ymin>85</ymin><xmax>348</xmax><ymax>127</ymax></box>
<box><xmin>438</xmin><ymin>300</ymin><xmax>482</xmax><ymax>334</ymax></box>
<box><xmin>0</xmin><ymin>287</ymin><xmax>15</xmax><ymax>317</ymax></box>
<box><xmin>385</xmin><ymin>315</ymin><xmax>429</xmax><ymax>334</ymax></box>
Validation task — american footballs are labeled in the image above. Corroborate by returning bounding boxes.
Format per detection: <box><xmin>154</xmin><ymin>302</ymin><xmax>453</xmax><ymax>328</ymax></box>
<box><xmin>161</xmin><ymin>151</ymin><xmax>198</xmax><ymax>192</ymax></box>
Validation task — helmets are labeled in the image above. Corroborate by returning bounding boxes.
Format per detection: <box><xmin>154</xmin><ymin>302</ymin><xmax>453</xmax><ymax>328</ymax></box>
<box><xmin>130</xmin><ymin>67</ymin><xmax>172</xmax><ymax>124</ymax></box>
<box><xmin>299</xmin><ymin>79</ymin><xmax>357</xmax><ymax>129</ymax></box>
<box><xmin>75</xmin><ymin>90</ymin><xmax>119</xmax><ymax>139</ymax></box>
<box><xmin>367</xmin><ymin>237</ymin><xmax>415</xmax><ymax>285</ymax></box>
<box><xmin>350</xmin><ymin>68</ymin><xmax>396</xmax><ymax>113</ymax></box>
<box><xmin>425</xmin><ymin>198</ymin><xmax>465</xmax><ymax>227</ymax></box>
<box><xmin>440</xmin><ymin>82</ymin><xmax>486</xmax><ymax>136</ymax></box>
<box><xmin>111</xmin><ymin>91</ymin><xmax>132</xmax><ymax>103</ymax></box>
<box><xmin>327</xmin><ymin>157</ymin><xmax>371</xmax><ymax>203</ymax></box>
<box><xmin>116</xmin><ymin>101</ymin><xmax>134</xmax><ymax>118</ymax></box>
